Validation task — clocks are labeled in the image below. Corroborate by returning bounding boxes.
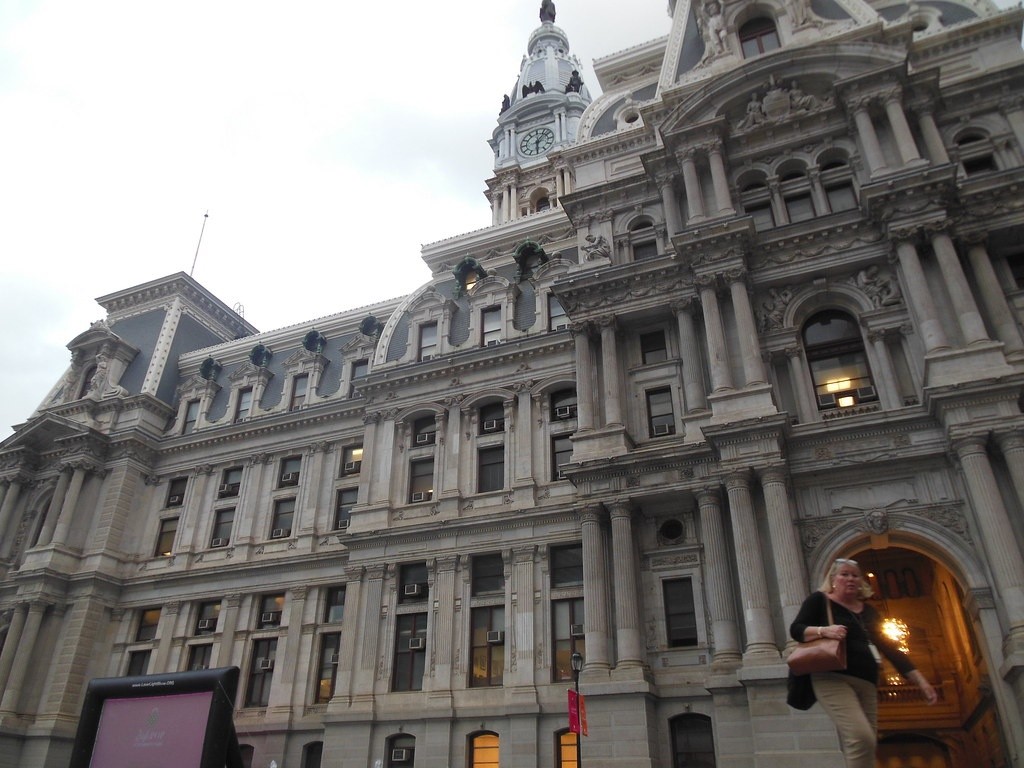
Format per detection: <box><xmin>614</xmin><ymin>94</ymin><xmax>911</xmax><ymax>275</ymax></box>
<box><xmin>520</xmin><ymin>128</ymin><xmax>554</xmax><ymax>155</ymax></box>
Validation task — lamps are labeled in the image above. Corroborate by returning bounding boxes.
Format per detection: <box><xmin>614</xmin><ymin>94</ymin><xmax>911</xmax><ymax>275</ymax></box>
<box><xmin>871</xmin><ymin>551</ymin><xmax>912</xmax><ymax>656</ymax></box>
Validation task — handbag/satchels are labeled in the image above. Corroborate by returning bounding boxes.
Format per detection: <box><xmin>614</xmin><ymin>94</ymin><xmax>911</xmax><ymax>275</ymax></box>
<box><xmin>787</xmin><ymin>592</ymin><xmax>847</xmax><ymax>676</ymax></box>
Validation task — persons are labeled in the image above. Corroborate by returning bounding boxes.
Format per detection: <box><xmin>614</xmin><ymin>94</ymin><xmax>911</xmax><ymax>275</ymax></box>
<box><xmin>59</xmin><ymin>362</ymin><xmax>82</xmax><ymax>402</ymax></box>
<box><xmin>581</xmin><ymin>235</ymin><xmax>611</xmax><ymax>263</ymax></box>
<box><xmin>499</xmin><ymin>95</ymin><xmax>510</xmax><ymax>115</ymax></box>
<box><xmin>565</xmin><ymin>70</ymin><xmax>583</xmax><ymax>93</ymax></box>
<box><xmin>858</xmin><ymin>265</ymin><xmax>900</xmax><ymax>308</ymax></box>
<box><xmin>84</xmin><ymin>353</ymin><xmax>108</xmax><ymax>399</ymax></box>
<box><xmin>869</xmin><ymin>511</ymin><xmax>885</xmax><ymax>530</ymax></box>
<box><xmin>736</xmin><ymin>81</ymin><xmax>835</xmax><ymax>131</ymax></box>
<box><xmin>761</xmin><ymin>287</ymin><xmax>793</xmax><ymax>334</ymax></box>
<box><xmin>782</xmin><ymin>0</ymin><xmax>822</xmax><ymax>35</ymax></box>
<box><xmin>700</xmin><ymin>0</ymin><xmax>730</xmax><ymax>59</ymax></box>
<box><xmin>790</xmin><ymin>558</ymin><xmax>938</xmax><ymax>768</ymax></box>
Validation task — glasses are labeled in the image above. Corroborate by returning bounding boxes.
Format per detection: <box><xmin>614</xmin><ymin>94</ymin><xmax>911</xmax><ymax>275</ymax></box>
<box><xmin>835</xmin><ymin>558</ymin><xmax>858</xmax><ymax>565</ymax></box>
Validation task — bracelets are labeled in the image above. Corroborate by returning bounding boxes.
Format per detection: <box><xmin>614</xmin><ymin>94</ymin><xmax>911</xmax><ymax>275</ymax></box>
<box><xmin>817</xmin><ymin>627</ymin><xmax>822</xmax><ymax>638</ymax></box>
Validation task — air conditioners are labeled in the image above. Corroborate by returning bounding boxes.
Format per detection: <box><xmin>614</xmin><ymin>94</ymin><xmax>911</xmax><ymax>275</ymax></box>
<box><xmin>568</xmin><ymin>623</ymin><xmax>584</xmax><ymax>637</ymax></box>
<box><xmin>219</xmin><ymin>483</ymin><xmax>232</xmax><ymax>494</ymax></box>
<box><xmin>236</xmin><ymin>325</ymin><xmax>572</xmax><ymax>445</ymax></box>
<box><xmin>653</xmin><ymin>423</ymin><xmax>670</xmax><ymax>436</ymax></box>
<box><xmin>212</xmin><ymin>538</ymin><xmax>227</xmax><ymax>547</ymax></box>
<box><xmin>338</xmin><ymin>519</ymin><xmax>349</xmax><ymax>528</ymax></box>
<box><xmin>194</xmin><ymin>665</ymin><xmax>208</xmax><ymax>672</ymax></box>
<box><xmin>487</xmin><ymin>631</ymin><xmax>505</xmax><ymax>644</ymax></box>
<box><xmin>282</xmin><ymin>473</ymin><xmax>297</xmax><ymax>484</ymax></box>
<box><xmin>345</xmin><ymin>461</ymin><xmax>357</xmax><ymax>472</ymax></box>
<box><xmin>273</xmin><ymin>529</ymin><xmax>288</xmax><ymax>537</ymax></box>
<box><xmin>818</xmin><ymin>385</ymin><xmax>877</xmax><ymax>407</ymax></box>
<box><xmin>262</xmin><ymin>613</ymin><xmax>277</xmax><ymax>622</ymax></box>
<box><xmin>412</xmin><ymin>492</ymin><xmax>424</xmax><ymax>502</ymax></box>
<box><xmin>405</xmin><ymin>583</ymin><xmax>422</xmax><ymax>596</ymax></box>
<box><xmin>409</xmin><ymin>638</ymin><xmax>426</xmax><ymax>650</ymax></box>
<box><xmin>199</xmin><ymin>619</ymin><xmax>215</xmax><ymax>630</ymax></box>
<box><xmin>261</xmin><ymin>659</ymin><xmax>274</xmax><ymax>671</ymax></box>
<box><xmin>170</xmin><ymin>496</ymin><xmax>181</xmax><ymax>504</ymax></box>
<box><xmin>392</xmin><ymin>748</ymin><xmax>410</xmax><ymax>762</ymax></box>
